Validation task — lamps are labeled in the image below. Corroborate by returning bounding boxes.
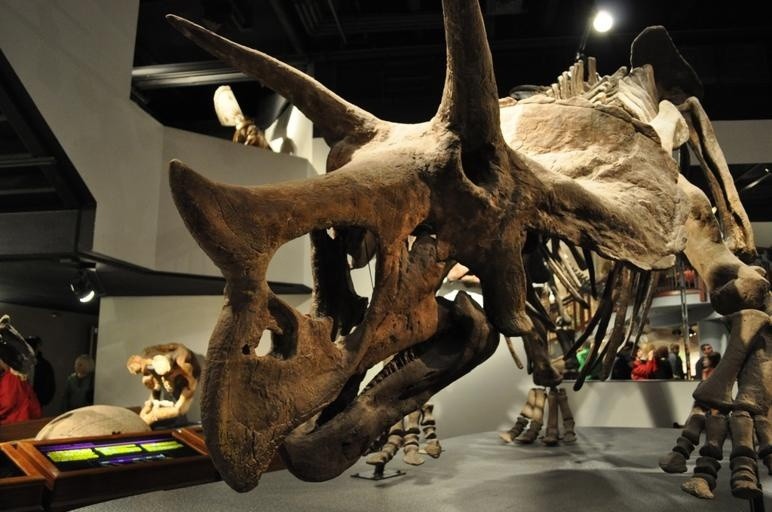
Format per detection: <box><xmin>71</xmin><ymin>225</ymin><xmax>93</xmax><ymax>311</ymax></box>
<box><xmin>67</xmin><ymin>269</ymin><xmax>108</xmax><ymax>304</ymax></box>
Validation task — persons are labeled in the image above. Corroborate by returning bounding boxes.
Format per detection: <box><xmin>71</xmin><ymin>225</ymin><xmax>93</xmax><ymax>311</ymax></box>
<box><xmin>0</xmin><ymin>335</ymin><xmax>95</xmax><ymax>425</ymax></box>
<box><xmin>576</xmin><ymin>338</ymin><xmax>721</xmax><ymax>381</ymax></box>
